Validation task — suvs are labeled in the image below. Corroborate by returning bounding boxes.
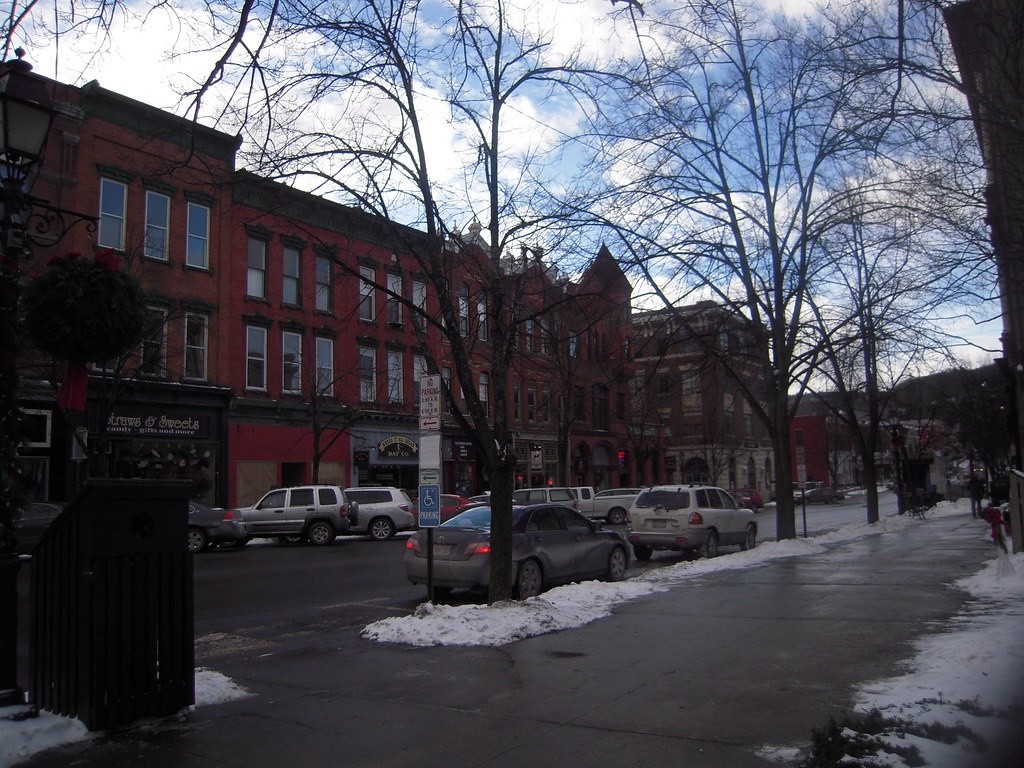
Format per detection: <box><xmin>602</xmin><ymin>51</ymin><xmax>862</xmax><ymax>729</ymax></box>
<box><xmin>209</xmin><ymin>485</ymin><xmax>359</xmax><ymax>553</ymax></box>
<box><xmin>344</xmin><ymin>487</ymin><xmax>415</xmax><ymax>540</ymax></box>
<box><xmin>627</xmin><ymin>481</ymin><xmax>757</xmax><ymax>558</ymax></box>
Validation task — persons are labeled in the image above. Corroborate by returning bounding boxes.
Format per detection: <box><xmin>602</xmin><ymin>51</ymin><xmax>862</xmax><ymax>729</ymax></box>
<box><xmin>199</xmin><ymin>465</ymin><xmax>213</xmax><ymax>504</ymax></box>
<box><xmin>969</xmin><ymin>470</ymin><xmax>984</xmax><ymax>519</ymax></box>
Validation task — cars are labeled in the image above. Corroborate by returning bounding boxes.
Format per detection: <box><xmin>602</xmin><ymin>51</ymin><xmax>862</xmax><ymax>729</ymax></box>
<box><xmin>411</xmin><ymin>487</ymin><xmax>649</xmax><ymax>530</ymax></box>
<box><xmin>795</xmin><ymin>487</ymin><xmax>844</xmax><ymax>506</ymax></box>
<box><xmin>725</xmin><ymin>487</ymin><xmax>762</xmax><ymax>508</ymax></box>
<box><xmin>406</xmin><ymin>503</ymin><xmax>632</xmax><ymax>600</ymax></box>
<box><xmin>9</xmin><ymin>501</ymin><xmax>67</xmax><ymax>559</ymax></box>
<box><xmin>189</xmin><ymin>499</ymin><xmax>249</xmax><ymax>551</ymax></box>
